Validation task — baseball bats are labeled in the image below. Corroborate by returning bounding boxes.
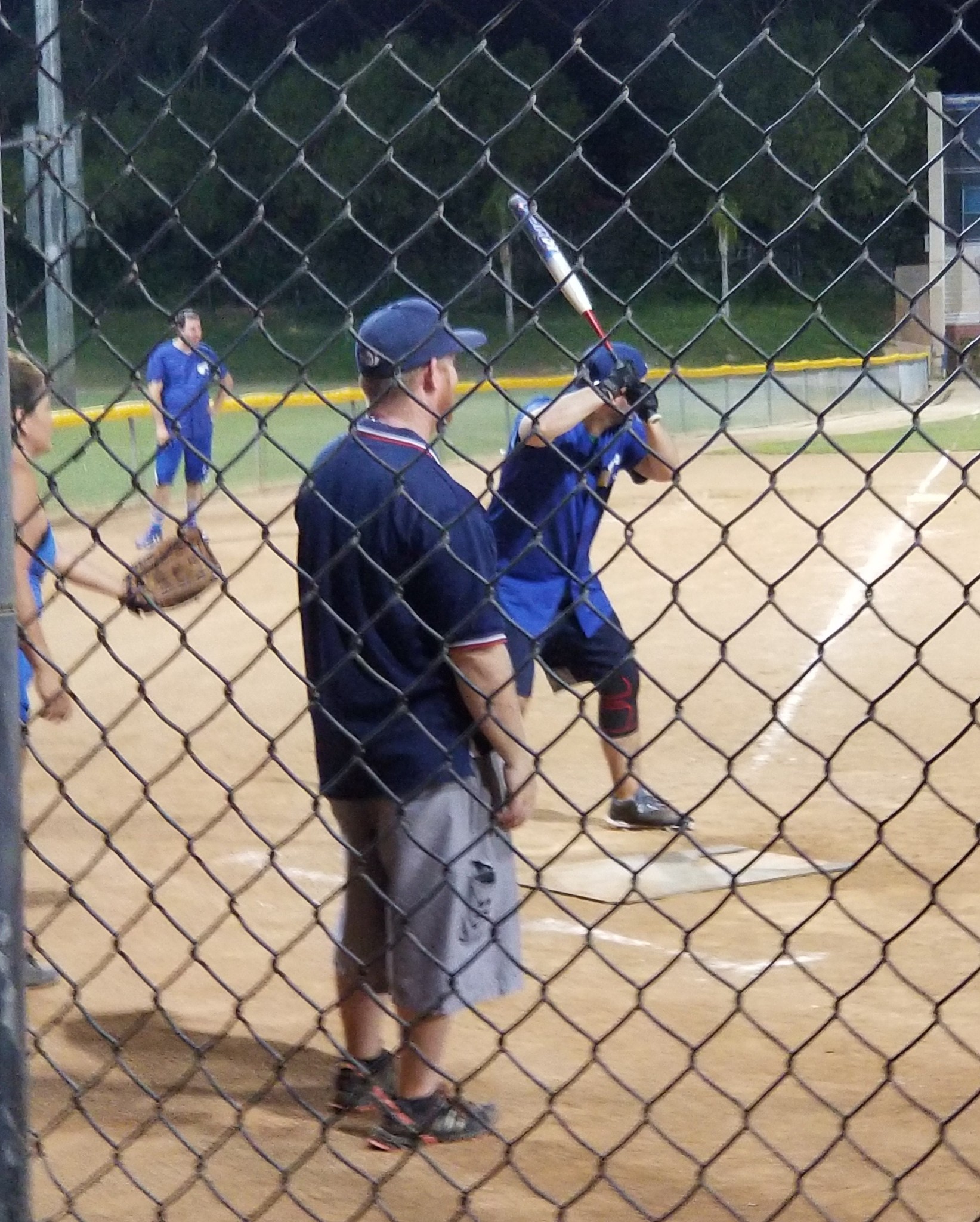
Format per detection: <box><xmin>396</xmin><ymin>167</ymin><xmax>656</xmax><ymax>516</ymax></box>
<box><xmin>505</xmin><ymin>192</ymin><xmax>664</xmax><ymax>424</ymax></box>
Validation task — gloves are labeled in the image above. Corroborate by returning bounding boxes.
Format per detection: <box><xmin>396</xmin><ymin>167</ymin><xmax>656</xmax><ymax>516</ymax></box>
<box><xmin>604</xmin><ymin>356</ymin><xmax>661</xmax><ymax>426</ymax></box>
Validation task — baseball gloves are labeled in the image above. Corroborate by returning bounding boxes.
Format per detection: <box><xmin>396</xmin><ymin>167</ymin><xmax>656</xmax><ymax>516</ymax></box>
<box><xmin>122</xmin><ymin>526</ymin><xmax>225</xmax><ymax>613</ymax></box>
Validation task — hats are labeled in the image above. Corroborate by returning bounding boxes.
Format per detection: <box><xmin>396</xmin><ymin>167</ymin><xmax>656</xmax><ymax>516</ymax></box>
<box><xmin>575</xmin><ymin>342</ymin><xmax>650</xmax><ymax>389</ymax></box>
<box><xmin>355</xmin><ymin>295</ymin><xmax>488</xmax><ymax>381</ymax></box>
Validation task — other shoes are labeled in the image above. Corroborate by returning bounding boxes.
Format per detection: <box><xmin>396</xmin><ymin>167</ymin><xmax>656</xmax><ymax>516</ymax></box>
<box><xmin>175</xmin><ymin>519</ymin><xmax>209</xmax><ymax>542</ymax></box>
<box><xmin>135</xmin><ymin>531</ymin><xmax>163</xmax><ymax>549</ymax></box>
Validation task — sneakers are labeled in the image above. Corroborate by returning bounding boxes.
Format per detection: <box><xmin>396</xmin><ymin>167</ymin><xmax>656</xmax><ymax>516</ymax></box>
<box><xmin>362</xmin><ymin>1075</ymin><xmax>501</xmax><ymax>1151</ymax></box>
<box><xmin>329</xmin><ymin>1046</ymin><xmax>405</xmax><ymax>1121</ymax></box>
<box><xmin>604</xmin><ymin>786</ymin><xmax>696</xmax><ymax>831</ymax></box>
<box><xmin>21</xmin><ymin>946</ymin><xmax>63</xmax><ymax>993</ymax></box>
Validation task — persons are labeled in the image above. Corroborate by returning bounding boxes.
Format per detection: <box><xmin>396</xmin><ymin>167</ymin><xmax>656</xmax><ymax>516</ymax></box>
<box><xmin>294</xmin><ymin>298</ymin><xmax>535</xmax><ymax>1153</ymax></box>
<box><xmin>9</xmin><ymin>347</ymin><xmax>222</xmax><ymax>989</ymax></box>
<box><xmin>487</xmin><ymin>343</ymin><xmax>691</xmax><ymax>829</ymax></box>
<box><xmin>136</xmin><ymin>308</ymin><xmax>234</xmax><ymax>548</ymax></box>
